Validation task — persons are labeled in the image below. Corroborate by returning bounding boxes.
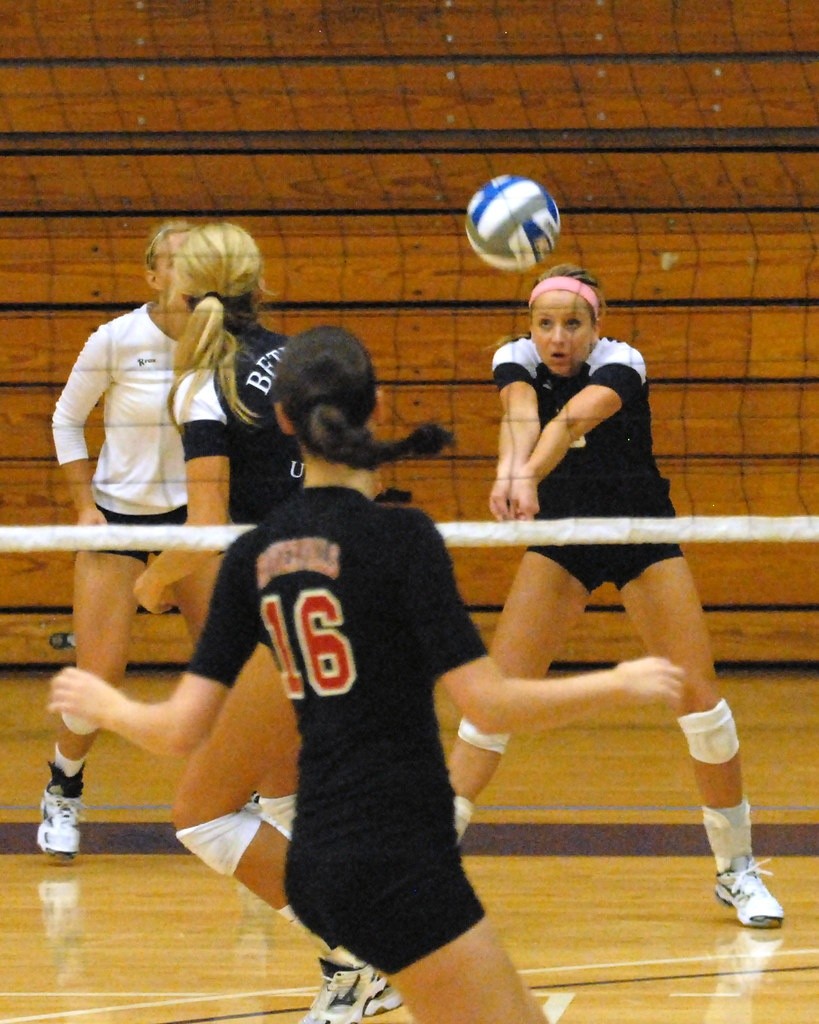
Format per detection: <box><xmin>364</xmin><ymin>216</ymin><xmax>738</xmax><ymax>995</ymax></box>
<box><xmin>447</xmin><ymin>264</ymin><xmax>784</xmax><ymax>929</ymax></box>
<box><xmin>36</xmin><ymin>221</ymin><xmax>231</xmax><ymax>856</ymax></box>
<box><xmin>131</xmin><ymin>223</ymin><xmax>405</xmax><ymax>1024</ymax></box>
<box><xmin>47</xmin><ymin>325</ymin><xmax>685</xmax><ymax>1024</ymax></box>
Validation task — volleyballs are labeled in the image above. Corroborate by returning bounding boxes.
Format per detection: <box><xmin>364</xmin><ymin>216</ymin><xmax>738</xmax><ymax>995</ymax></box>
<box><xmin>464</xmin><ymin>174</ymin><xmax>562</xmax><ymax>273</ymax></box>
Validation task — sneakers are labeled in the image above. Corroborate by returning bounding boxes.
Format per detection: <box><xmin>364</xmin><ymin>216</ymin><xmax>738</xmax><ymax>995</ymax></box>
<box><xmin>37</xmin><ymin>793</ymin><xmax>87</xmax><ymax>858</ymax></box>
<box><xmin>714</xmin><ymin>854</ymin><xmax>784</xmax><ymax>927</ymax></box>
<box><xmin>298</xmin><ymin>956</ymin><xmax>403</xmax><ymax>1024</ymax></box>
<box><xmin>718</xmin><ymin>928</ymin><xmax>782</xmax><ymax>976</ymax></box>
<box><xmin>39</xmin><ymin>875</ymin><xmax>79</xmax><ymax>929</ymax></box>
